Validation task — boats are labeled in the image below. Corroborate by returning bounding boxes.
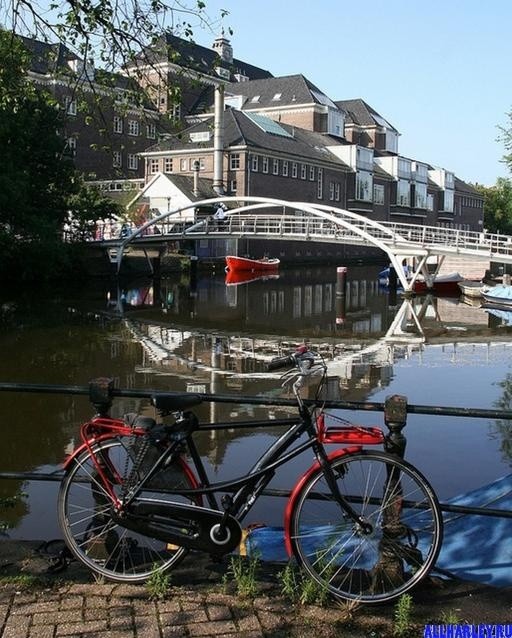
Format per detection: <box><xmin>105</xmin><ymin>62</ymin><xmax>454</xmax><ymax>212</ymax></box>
<box><xmin>213</xmin><ymin>329</ymin><xmax>362</xmax><ymax>370</ymax></box>
<box><xmin>222</xmin><ymin>272</ymin><xmax>280</xmax><ymax>289</ymax></box>
<box><xmin>456</xmin><ymin>279</ymin><xmax>512</xmax><ymax>307</ymax></box>
<box><xmin>376</xmin><ymin>265</ymin><xmax>460</xmax><ymax>290</ymax></box>
<box><xmin>226</xmin><ymin>254</ymin><xmax>280</xmax><ymax>272</ymax></box>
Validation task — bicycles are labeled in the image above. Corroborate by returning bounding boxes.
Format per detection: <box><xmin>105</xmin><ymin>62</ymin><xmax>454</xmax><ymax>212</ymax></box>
<box><xmin>59</xmin><ymin>335</ymin><xmax>447</xmax><ymax>608</ymax></box>
